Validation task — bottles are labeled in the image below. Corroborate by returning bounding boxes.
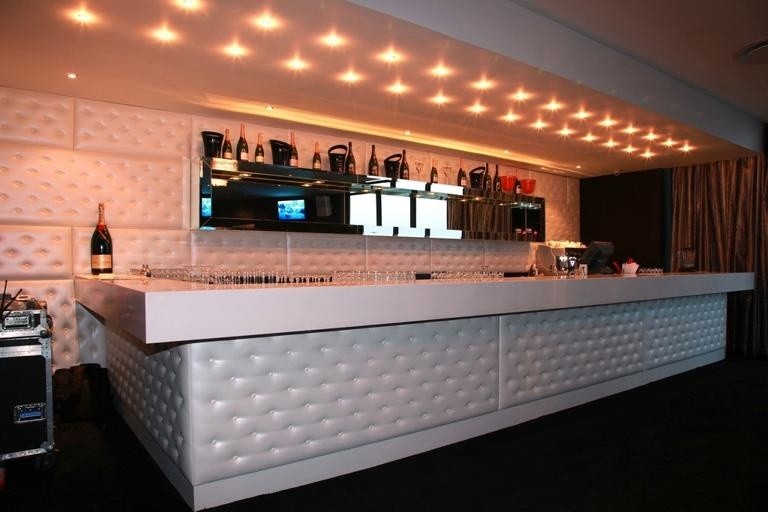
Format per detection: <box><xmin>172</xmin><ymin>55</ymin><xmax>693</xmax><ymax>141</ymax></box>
<box><xmin>287</xmin><ymin>128</ymin><xmax>299</xmax><ymax>165</ymax></box>
<box><xmin>236</xmin><ymin>122</ymin><xmax>249</xmax><ymax>161</ymax></box>
<box><xmin>311</xmin><ymin>141</ymin><xmax>321</xmax><ymax>170</ymax></box>
<box><xmin>220</xmin><ymin>128</ymin><xmax>234</xmax><ymax>160</ymax></box>
<box><xmin>398</xmin><ymin>147</ymin><xmax>520</xmax><ymax>196</ymax></box>
<box><xmin>252</xmin><ymin>132</ymin><xmax>265</xmax><ymax>163</ymax></box>
<box><xmin>344</xmin><ymin>141</ymin><xmax>356</xmax><ymax>173</ymax></box>
<box><xmin>367</xmin><ymin>143</ymin><xmax>380</xmax><ymax>176</ymax></box>
<box><xmin>89</xmin><ymin>201</ymin><xmax>113</xmax><ymax>275</ymax></box>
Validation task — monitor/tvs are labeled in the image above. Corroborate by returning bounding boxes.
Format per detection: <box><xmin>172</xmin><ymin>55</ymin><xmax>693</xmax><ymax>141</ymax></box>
<box><xmin>577</xmin><ymin>241</ymin><xmax>615</xmax><ymax>275</ymax></box>
<box><xmin>275</xmin><ymin>199</ymin><xmax>310</xmax><ymax>221</ymax></box>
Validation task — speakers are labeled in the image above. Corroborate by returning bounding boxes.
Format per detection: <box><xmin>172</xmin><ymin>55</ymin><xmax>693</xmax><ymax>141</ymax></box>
<box><xmin>0</xmin><ymin>310</ymin><xmax>55</xmax><ymax>462</ymax></box>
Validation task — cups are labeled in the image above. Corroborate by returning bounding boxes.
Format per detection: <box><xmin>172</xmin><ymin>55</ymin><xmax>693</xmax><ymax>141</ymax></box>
<box><xmin>130</xmin><ymin>265</ymin><xmax>415</xmax><ymax>286</ymax></box>
<box><xmin>558</xmin><ymin>268</ymin><xmax>567</xmax><ymax>280</ymax></box>
<box><xmin>638</xmin><ymin>268</ymin><xmax>663</xmax><ymax>274</ymax></box>
<box><xmin>428</xmin><ymin>269</ymin><xmax>504</xmax><ymax>281</ymax></box>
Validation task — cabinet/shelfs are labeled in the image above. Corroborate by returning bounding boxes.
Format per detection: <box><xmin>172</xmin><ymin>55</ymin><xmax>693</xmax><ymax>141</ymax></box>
<box><xmin>191</xmin><ymin>157</ymin><xmax>546</xmax><ymax>242</ymax></box>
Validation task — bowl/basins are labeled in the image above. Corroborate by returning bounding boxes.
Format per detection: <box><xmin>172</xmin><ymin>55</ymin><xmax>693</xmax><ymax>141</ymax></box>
<box><xmin>518</xmin><ymin>177</ymin><xmax>536</xmax><ymax>194</ymax></box>
<box><xmin>622</xmin><ymin>263</ymin><xmax>639</xmax><ymax>275</ymax></box>
